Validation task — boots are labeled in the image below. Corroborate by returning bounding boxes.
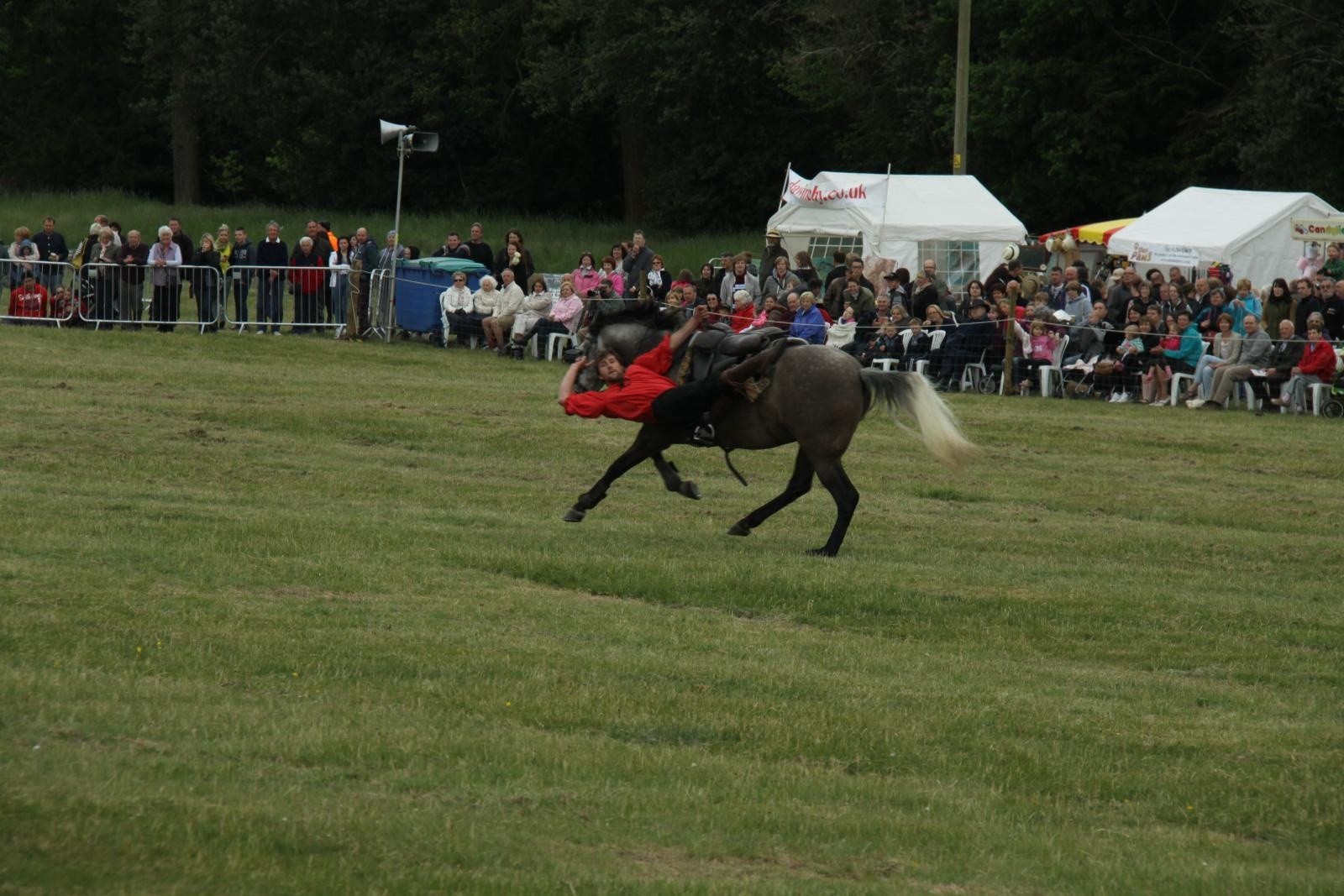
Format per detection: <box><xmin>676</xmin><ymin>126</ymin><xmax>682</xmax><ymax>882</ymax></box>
<box><xmin>720</xmin><ymin>338</ymin><xmax>788</xmax><ymax>386</ymax></box>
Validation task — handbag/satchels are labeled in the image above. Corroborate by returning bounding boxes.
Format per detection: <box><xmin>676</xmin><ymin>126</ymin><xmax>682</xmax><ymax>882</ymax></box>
<box><xmin>1094</xmin><ymin>362</ymin><xmax>1114</xmax><ymax>375</ymax></box>
<box><xmin>1205</xmin><ymin>331</ymin><xmax>1216</xmax><ymax>341</ymax></box>
<box><xmin>289</xmin><ymin>286</ymin><xmax>293</xmax><ymax>294</ymax></box>
<box><xmin>71</xmin><ymin>238</ymin><xmax>88</xmax><ymax>270</ymax></box>
<box><xmin>89</xmin><ymin>270</ymin><xmax>98</xmax><ymax>279</ymax></box>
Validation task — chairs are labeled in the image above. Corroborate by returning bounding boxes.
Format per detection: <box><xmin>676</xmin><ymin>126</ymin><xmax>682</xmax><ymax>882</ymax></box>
<box><xmin>823</xmin><ymin>322</ymin><xmax>1343</xmax><ymax>416</ymax></box>
<box><xmin>440</xmin><ymin>291</ymin><xmax>584</xmax><ymax>362</ymax></box>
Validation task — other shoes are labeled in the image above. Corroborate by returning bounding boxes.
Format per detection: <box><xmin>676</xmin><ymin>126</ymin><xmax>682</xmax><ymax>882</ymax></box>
<box><xmin>142</xmin><ymin>323</ymin><xmax>281</xmax><ymax>335</ymax></box>
<box><xmin>939</xmin><ymin>378</ymin><xmax>1292</xmax><ymax>419</ymax></box>
<box><xmin>445</xmin><ymin>335</ymin><xmax>528</xmax><ymax>360</ymax></box>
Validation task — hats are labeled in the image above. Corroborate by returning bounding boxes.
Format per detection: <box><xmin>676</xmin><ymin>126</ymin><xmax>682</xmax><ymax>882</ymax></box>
<box><xmin>891</xmin><ymin>268</ymin><xmax>910</xmax><ymax>283</ymax></box>
<box><xmin>969</xmin><ymin>299</ymin><xmax>984</xmax><ymax>308</ymax></box>
<box><xmin>1002</xmin><ymin>243</ymin><xmax>1020</xmax><ymax>261</ymax></box>
<box><xmin>1053</xmin><ymin>238</ymin><xmax>1062</xmax><ymax>251</ymax></box>
<box><xmin>1327</xmin><ymin>242</ymin><xmax>1339</xmax><ymax>252</ymax></box>
<box><xmin>1046</xmin><ymin>238</ymin><xmax>1053</xmax><ymax>252</ymax></box>
<box><xmin>884</xmin><ymin>274</ymin><xmax>896</xmax><ymax>279</ymax></box>
<box><xmin>1022</xmin><ymin>274</ymin><xmax>1040</xmax><ymax>297</ymax></box>
<box><xmin>865</xmin><ymin>332</ymin><xmax>882</xmax><ymax>343</ymax></box>
<box><xmin>1062</xmin><ymin>234</ymin><xmax>1076</xmax><ymax>251</ymax></box>
<box><xmin>387</xmin><ymin>230</ymin><xmax>396</xmax><ymax>236</ymax></box>
<box><xmin>719</xmin><ymin>252</ymin><xmax>734</xmax><ymax>260</ymax></box>
<box><xmin>95</xmin><ymin>215</ymin><xmax>107</xmax><ymax>223</ymax></box>
<box><xmin>762</xmin><ymin>230</ymin><xmax>781</xmax><ymax>238</ymax></box>
<box><xmin>1110</xmin><ymin>268</ymin><xmax>1125</xmax><ymax>280</ymax></box>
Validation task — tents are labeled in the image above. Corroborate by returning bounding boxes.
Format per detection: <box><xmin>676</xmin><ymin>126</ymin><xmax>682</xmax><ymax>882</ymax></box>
<box><xmin>766</xmin><ymin>162</ymin><xmax>1028</xmax><ymax>306</ymax></box>
<box><xmin>1111</xmin><ymin>187</ymin><xmax>1344</xmax><ymax>293</ymax></box>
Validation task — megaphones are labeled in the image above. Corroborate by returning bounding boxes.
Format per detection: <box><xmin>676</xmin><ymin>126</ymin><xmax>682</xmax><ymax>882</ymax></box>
<box><xmin>380</xmin><ymin>119</ymin><xmax>409</xmax><ymax>144</ymax></box>
<box><xmin>404</xmin><ymin>130</ymin><xmax>439</xmax><ymax>152</ymax></box>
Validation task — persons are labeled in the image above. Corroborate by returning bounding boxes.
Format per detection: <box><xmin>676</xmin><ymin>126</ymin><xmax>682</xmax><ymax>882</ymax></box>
<box><xmin>8</xmin><ymin>215</ymin><xmax>420</xmax><ymax>338</ymax></box>
<box><xmin>432</xmin><ymin>222</ymin><xmax>1344</xmax><ymax>426</ymax></box>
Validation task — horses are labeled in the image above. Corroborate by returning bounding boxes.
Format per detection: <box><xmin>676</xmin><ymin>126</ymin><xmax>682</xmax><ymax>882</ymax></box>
<box><xmin>562</xmin><ymin>300</ymin><xmax>989</xmax><ymax>556</ymax></box>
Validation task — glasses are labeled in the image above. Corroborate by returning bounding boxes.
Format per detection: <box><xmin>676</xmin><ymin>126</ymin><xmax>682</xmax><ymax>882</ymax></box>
<box><xmin>582</xmin><ymin>252</ymin><xmax>591</xmax><ymax>254</ymax></box>
<box><xmin>454</xmin><ymin>279</ymin><xmax>464</xmax><ymax>282</ymax></box>
<box><xmin>652</xmin><ymin>262</ymin><xmax>661</xmax><ymax>264</ymax></box>
<box><xmin>1309</xmin><ymin>319</ymin><xmax>1321</xmax><ymax>323</ymax></box>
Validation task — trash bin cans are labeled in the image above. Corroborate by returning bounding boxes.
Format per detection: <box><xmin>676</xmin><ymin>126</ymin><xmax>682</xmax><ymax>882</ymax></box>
<box><xmin>392</xmin><ymin>257</ymin><xmax>493</xmax><ymax>346</ymax></box>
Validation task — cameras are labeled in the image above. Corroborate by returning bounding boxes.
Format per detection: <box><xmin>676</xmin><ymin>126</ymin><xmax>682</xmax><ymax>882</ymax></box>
<box><xmin>587</xmin><ymin>288</ymin><xmax>601</xmax><ymax>297</ymax></box>
